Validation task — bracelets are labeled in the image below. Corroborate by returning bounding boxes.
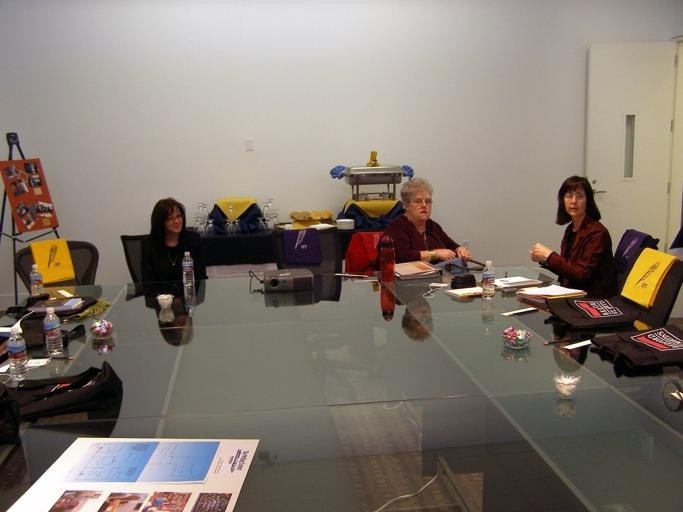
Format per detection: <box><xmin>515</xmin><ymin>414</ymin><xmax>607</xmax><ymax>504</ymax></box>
<box><xmin>428</xmin><ymin>247</ymin><xmax>439</xmax><ymax>262</ymax></box>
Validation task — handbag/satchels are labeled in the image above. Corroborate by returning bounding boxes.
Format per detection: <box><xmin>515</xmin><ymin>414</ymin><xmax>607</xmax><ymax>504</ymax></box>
<box><xmin>5</xmin><ymin>294</ymin><xmax>97</xmax><ymax>320</ymax></box>
<box><xmin>8</xmin><ymin>362</ymin><xmax>123</xmax><ymax>419</ymax></box>
<box><xmin>590</xmin><ymin>325</ymin><xmax>683</xmax><ymax>369</ymax></box>
<box><xmin>544</xmin><ymin>295</ymin><xmax>639</xmax><ymax>332</ymax></box>
<box><xmin>19</xmin><ymin>320</ymin><xmax>86</xmax><ymax>348</ymax></box>
<box><xmin>423</xmin><ymin>259</ymin><xmax>486</xmax><ymax>274</ymax></box>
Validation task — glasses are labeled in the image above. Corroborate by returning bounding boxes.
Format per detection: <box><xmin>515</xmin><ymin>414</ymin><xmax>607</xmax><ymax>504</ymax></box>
<box><xmin>408</xmin><ymin>198</ymin><xmax>432</xmax><ymax>207</ymax></box>
<box><xmin>166</xmin><ymin>213</ymin><xmax>184</xmax><ymax>220</ymax></box>
<box><xmin>564</xmin><ymin>194</ymin><xmax>586</xmax><ymax>201</ymax></box>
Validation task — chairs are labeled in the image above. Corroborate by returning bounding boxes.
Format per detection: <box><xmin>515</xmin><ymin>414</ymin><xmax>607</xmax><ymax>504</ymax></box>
<box><xmin>617</xmin><ymin>246</ymin><xmax>683</xmax><ymax>329</ymax></box>
<box><xmin>612</xmin><ymin>228</ymin><xmax>662</xmax><ymax>295</ymax></box>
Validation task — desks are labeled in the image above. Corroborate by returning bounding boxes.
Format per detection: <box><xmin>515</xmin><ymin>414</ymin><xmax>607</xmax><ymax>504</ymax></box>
<box><xmin>1</xmin><ymin>253</ymin><xmax>681</xmax><ymax>511</ymax></box>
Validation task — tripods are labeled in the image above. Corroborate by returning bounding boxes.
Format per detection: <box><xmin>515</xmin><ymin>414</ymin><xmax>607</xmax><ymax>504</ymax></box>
<box><xmin>0</xmin><ymin>132</ymin><xmax>80</xmax><ymax>307</ymax></box>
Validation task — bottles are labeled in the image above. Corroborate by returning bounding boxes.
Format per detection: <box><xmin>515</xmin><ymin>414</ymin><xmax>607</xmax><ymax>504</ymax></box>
<box><xmin>181</xmin><ymin>251</ymin><xmax>195</xmax><ymax>287</ymax></box>
<box><xmin>6</xmin><ymin>327</ymin><xmax>29</xmax><ymax>380</ymax></box>
<box><xmin>480</xmin><ymin>260</ymin><xmax>494</xmax><ymax>300</ymax></box>
<box><xmin>380</xmin><ymin>235</ymin><xmax>394</xmax><ymax>281</ymax></box>
<box><xmin>42</xmin><ymin>307</ymin><xmax>63</xmax><ymax>356</ymax></box>
<box><xmin>29</xmin><ymin>264</ymin><xmax>43</xmax><ymax>297</ymax></box>
<box><xmin>380</xmin><ymin>281</ymin><xmax>394</xmax><ymax>322</ymax></box>
<box><xmin>183</xmin><ymin>287</ymin><xmax>196</xmax><ymax>318</ymax></box>
<box><xmin>481</xmin><ymin>300</ymin><xmax>494</xmax><ymax>336</ymax></box>
<box><xmin>47</xmin><ymin>357</ymin><xmax>64</xmax><ymax>378</ymax></box>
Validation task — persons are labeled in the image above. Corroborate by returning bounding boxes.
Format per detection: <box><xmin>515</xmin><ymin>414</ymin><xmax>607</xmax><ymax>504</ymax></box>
<box><xmin>378</xmin><ymin>274</ymin><xmax>443</xmax><ymax>343</ymax></box>
<box><xmin>139</xmin><ymin>197</ymin><xmax>208</xmax><ymax>281</ymax></box>
<box><xmin>530</xmin><ymin>175</ymin><xmax>614</xmax><ymax>288</ymax></box>
<box><xmin>145</xmin><ymin>492</ymin><xmax>172</xmax><ymax>512</ymax></box>
<box><xmin>378</xmin><ymin>178</ymin><xmax>472</xmax><ymax>273</ymax></box>
<box><xmin>199</xmin><ymin>494</ymin><xmax>227</xmax><ymax>512</ymax></box>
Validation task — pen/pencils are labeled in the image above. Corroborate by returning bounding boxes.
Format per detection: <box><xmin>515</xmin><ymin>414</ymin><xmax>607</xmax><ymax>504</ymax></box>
<box><xmin>32</xmin><ymin>355</ymin><xmax>75</xmax><ymax>360</ymax></box>
<box><xmin>504</xmin><ymin>271</ymin><xmax>508</xmax><ymax>278</ymax></box>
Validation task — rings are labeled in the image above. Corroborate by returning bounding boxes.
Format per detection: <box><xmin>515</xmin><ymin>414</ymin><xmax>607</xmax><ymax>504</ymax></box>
<box><xmin>467</xmin><ymin>254</ymin><xmax>470</xmax><ymax>258</ymax></box>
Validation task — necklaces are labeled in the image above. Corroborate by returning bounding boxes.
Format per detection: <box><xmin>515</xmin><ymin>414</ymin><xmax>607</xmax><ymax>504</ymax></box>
<box><xmin>163</xmin><ymin>243</ymin><xmax>183</xmax><ymax>268</ymax></box>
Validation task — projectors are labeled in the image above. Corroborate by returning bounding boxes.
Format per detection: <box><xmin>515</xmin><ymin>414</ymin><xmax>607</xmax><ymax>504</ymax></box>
<box><xmin>263</xmin><ymin>268</ymin><xmax>314</xmax><ymax>291</ymax></box>
<box><xmin>264</xmin><ymin>289</ymin><xmax>316</xmax><ymax>308</ymax></box>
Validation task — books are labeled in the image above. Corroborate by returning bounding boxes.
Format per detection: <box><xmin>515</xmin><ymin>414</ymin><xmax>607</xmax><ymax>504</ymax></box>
<box><xmin>392</xmin><ymin>262</ymin><xmax>439</xmax><ymax>282</ymax></box>
<box><xmin>514</xmin><ymin>284</ymin><xmax>587</xmax><ymax>306</ymax></box>
